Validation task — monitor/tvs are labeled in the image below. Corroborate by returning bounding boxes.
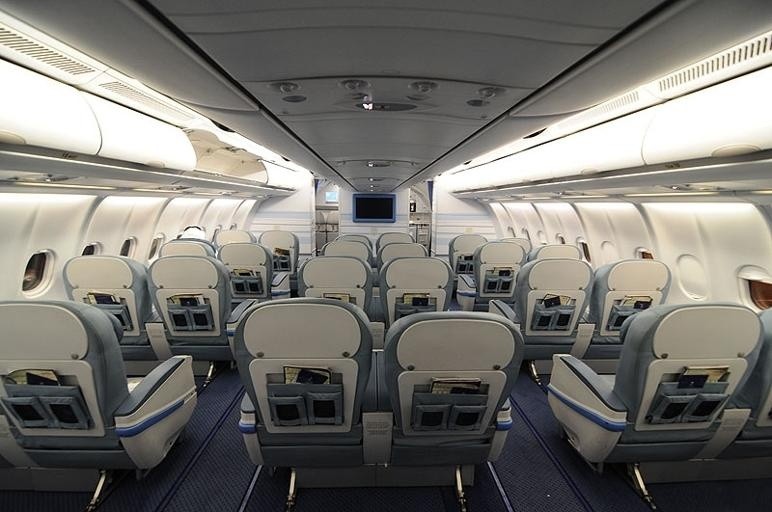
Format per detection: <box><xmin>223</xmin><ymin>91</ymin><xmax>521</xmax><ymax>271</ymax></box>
<box><xmin>351</xmin><ymin>193</ymin><xmax>396</xmax><ymax>223</ymax></box>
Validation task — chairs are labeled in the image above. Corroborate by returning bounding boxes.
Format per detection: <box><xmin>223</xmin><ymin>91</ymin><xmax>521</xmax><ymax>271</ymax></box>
<box><xmin>321</xmin><ymin>240</ymin><xmax>376</xmax><ymax>287</ymax></box>
<box><xmin>258</xmin><ymin>230</ymin><xmax>308</xmax><ymax>290</ymax></box>
<box><xmin>334</xmin><ymin>234</ymin><xmax>376</xmax><ymax>261</ymax></box>
<box><xmin>527</xmin><ymin>244</ymin><xmax>583</xmax><ymax>263</ymax></box>
<box><xmin>232</xmin><ymin>297</ymin><xmax>377</xmax><ymax>511</ymax></box>
<box><xmin>1</xmin><ymin>299</ymin><xmax>199</xmax><ymax>512</ymax></box>
<box><xmin>61</xmin><ymin>254</ymin><xmax>159</xmax><ymax>377</ymax></box>
<box><xmin>377</xmin><ymin>311</ymin><xmax>525</xmax><ymax>511</ymax></box>
<box><xmin>376</xmin><ymin>242</ymin><xmax>429</xmax><ymax>284</ymax></box>
<box><xmin>582</xmin><ymin>258</ymin><xmax>672</xmax><ymax>375</ymax></box>
<box><xmin>214</xmin><ymin>229</ymin><xmax>257</xmax><ymax>251</ymax></box>
<box><xmin>456</xmin><ymin>242</ymin><xmax>529</xmax><ymax>312</ymax></box>
<box><xmin>546</xmin><ymin>303</ymin><xmax>764</xmax><ymax>512</ymax></box>
<box><xmin>499</xmin><ymin>237</ymin><xmax>532</xmax><ymax>264</ymax></box>
<box><xmin>376</xmin><ymin>257</ymin><xmax>453</xmax><ymax>331</ymax></box>
<box><xmin>217</xmin><ymin>243</ymin><xmax>290</xmax><ymax>308</ymax></box>
<box><xmin>298</xmin><ymin>256</ymin><xmax>376</xmax><ymax>322</ymax></box>
<box><xmin>488</xmin><ymin>258</ymin><xmax>596</xmax><ymax>388</ymax></box>
<box><xmin>375</xmin><ymin>232</ymin><xmax>416</xmax><ymax>263</ymax></box>
<box><xmin>158</xmin><ymin>238</ymin><xmax>217</xmax><ymax>260</ymax></box>
<box><xmin>147</xmin><ymin>254</ymin><xmax>260</xmax><ymax>384</ymax></box>
<box><xmin>732</xmin><ymin>306</ymin><xmax>771</xmax><ymax>464</ymax></box>
<box><xmin>448</xmin><ymin>234</ymin><xmax>489</xmax><ymax>282</ymax></box>
